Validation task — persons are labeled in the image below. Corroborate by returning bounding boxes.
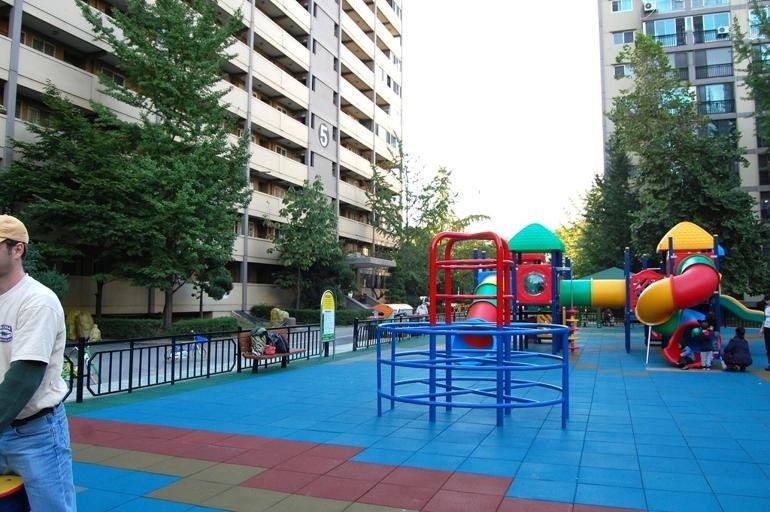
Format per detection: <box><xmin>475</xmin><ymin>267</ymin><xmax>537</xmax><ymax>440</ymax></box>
<box><xmin>601</xmin><ymin>305</ymin><xmax>616</xmax><ymax>326</ymax></box>
<box><xmin>415</xmin><ymin>302</ymin><xmax>467</xmax><ymax>322</ymax></box>
<box><xmin>671</xmin><ymin>293</ymin><xmax>770</xmax><ymax>373</ymax></box>
<box><xmin>0</xmin><ymin>213</ymin><xmax>79</xmax><ymax>512</ymax></box>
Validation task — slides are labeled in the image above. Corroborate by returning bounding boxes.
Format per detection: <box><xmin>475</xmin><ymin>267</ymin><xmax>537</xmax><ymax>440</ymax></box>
<box><xmin>634</xmin><ymin>254</ymin><xmax>722</xmax><ymax>368</ymax></box>
<box><xmin>451</xmin><ymin>275</ymin><xmax>504</xmax><ymax>365</ymax></box>
<box><xmin>720</xmin><ymin>294</ymin><xmax>765</xmax><ymax>323</ymax></box>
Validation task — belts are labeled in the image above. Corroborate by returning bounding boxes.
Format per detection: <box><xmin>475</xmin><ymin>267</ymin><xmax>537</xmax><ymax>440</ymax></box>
<box><xmin>10</xmin><ymin>399</ymin><xmax>61</xmax><ymax>428</ymax></box>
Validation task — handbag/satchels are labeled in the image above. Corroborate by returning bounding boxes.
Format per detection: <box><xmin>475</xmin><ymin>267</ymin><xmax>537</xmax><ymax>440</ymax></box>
<box><xmin>264</xmin><ymin>341</ymin><xmax>276</xmax><ymax>354</ymax></box>
<box><xmin>249</xmin><ymin>327</ymin><xmax>268</xmax><ymax>357</ymax></box>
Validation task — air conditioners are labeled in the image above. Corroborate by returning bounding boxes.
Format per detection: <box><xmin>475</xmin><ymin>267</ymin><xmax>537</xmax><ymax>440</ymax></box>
<box><xmin>717</xmin><ymin>26</ymin><xmax>729</xmax><ymax>35</ymax></box>
<box><xmin>643</xmin><ymin>2</ymin><xmax>657</xmax><ymax>13</ymax></box>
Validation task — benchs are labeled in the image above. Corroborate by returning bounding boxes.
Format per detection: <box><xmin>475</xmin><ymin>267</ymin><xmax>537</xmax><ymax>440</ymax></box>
<box><xmin>238</xmin><ymin>328</ymin><xmax>307</xmax><ymax>374</ymax></box>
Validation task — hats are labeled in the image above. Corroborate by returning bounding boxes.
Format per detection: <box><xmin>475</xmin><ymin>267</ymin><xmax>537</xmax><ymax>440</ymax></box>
<box><xmin>0</xmin><ymin>213</ymin><xmax>30</xmax><ymax>245</ymax></box>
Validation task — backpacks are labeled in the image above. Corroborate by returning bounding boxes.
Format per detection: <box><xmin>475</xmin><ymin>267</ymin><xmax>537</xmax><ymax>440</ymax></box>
<box><xmin>268</xmin><ymin>332</ymin><xmax>288</xmax><ymax>352</ymax></box>
